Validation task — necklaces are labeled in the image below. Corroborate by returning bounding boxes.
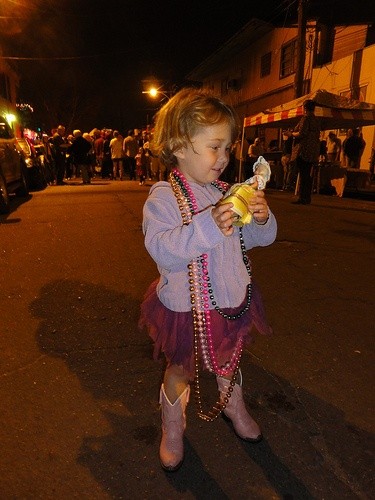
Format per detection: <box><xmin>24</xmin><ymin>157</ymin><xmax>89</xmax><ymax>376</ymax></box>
<box><xmin>165</xmin><ymin>167</ymin><xmax>254</xmax><ymax>423</ymax></box>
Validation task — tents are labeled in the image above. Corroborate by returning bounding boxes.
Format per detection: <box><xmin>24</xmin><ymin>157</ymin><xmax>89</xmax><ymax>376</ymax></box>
<box><xmin>238</xmin><ymin>89</ymin><xmax>375</xmax><ymax>198</ymax></box>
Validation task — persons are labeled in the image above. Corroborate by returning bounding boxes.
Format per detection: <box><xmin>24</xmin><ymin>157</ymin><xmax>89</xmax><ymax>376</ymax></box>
<box><xmin>217</xmin><ymin>155</ymin><xmax>272</xmax><ymax>226</ymax></box>
<box><xmin>20</xmin><ymin>123</ymin><xmax>366</xmax><ymax>187</ymax></box>
<box><xmin>140</xmin><ymin>86</ymin><xmax>278</xmax><ymax>472</ymax></box>
<box><xmin>289</xmin><ymin>98</ymin><xmax>321</xmax><ymax>206</ymax></box>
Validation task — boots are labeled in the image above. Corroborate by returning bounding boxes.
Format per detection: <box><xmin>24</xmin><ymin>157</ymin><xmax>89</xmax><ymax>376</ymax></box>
<box><xmin>216</xmin><ymin>368</ymin><xmax>263</xmax><ymax>440</ymax></box>
<box><xmin>158</xmin><ymin>383</ymin><xmax>190</xmax><ymax>470</ymax></box>
